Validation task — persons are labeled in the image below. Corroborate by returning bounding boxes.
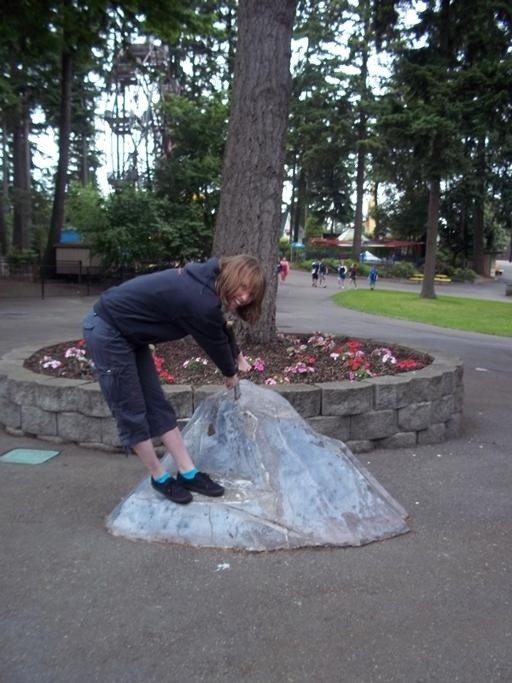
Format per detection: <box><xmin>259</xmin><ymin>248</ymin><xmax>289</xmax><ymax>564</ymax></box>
<box><xmin>312</xmin><ymin>258</ymin><xmax>328</xmax><ymax>287</ymax></box>
<box><xmin>279</xmin><ymin>257</ymin><xmax>290</xmax><ymax>284</ymax></box>
<box><xmin>336</xmin><ymin>259</ymin><xmax>347</xmax><ymax>289</ymax></box>
<box><xmin>367</xmin><ymin>264</ymin><xmax>378</xmax><ymax>290</ymax></box>
<box><xmin>346</xmin><ymin>263</ymin><xmax>357</xmax><ymax>288</ymax></box>
<box><xmin>81</xmin><ymin>254</ymin><xmax>268</xmax><ymax>504</ymax></box>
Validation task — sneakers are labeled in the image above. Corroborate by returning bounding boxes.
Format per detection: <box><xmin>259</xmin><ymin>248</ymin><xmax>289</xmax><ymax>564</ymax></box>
<box><xmin>150</xmin><ymin>474</ymin><xmax>192</xmax><ymax>504</ymax></box>
<box><xmin>176</xmin><ymin>471</ymin><xmax>225</xmax><ymax>496</ymax></box>
